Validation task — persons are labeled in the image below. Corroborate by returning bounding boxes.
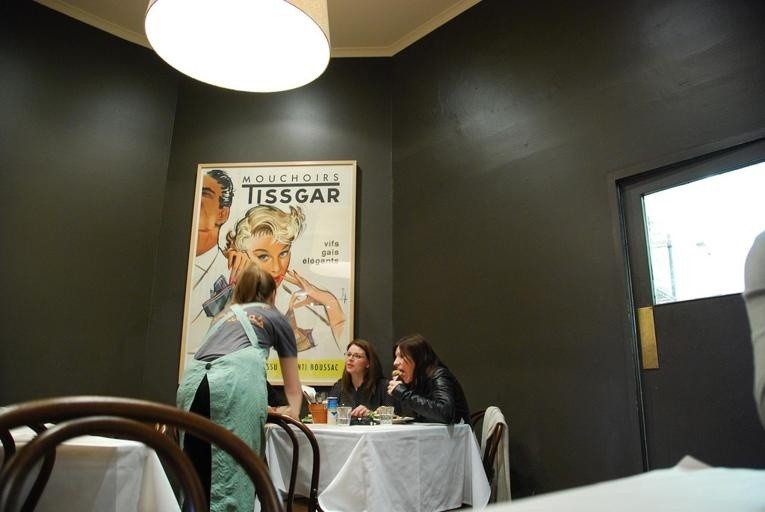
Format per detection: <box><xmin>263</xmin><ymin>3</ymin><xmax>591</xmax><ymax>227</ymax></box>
<box><xmin>330</xmin><ymin>338</ymin><xmax>386</xmax><ymax>417</ymax></box>
<box><xmin>210</xmin><ymin>204</ymin><xmax>346</xmax><ymax>352</ymax></box>
<box><xmin>177</xmin><ymin>263</ymin><xmax>302</xmax><ymax>512</ymax></box>
<box><xmin>186</xmin><ymin>170</ymin><xmax>237</xmax><ymax>367</ymax></box>
<box><xmin>386</xmin><ymin>335</ymin><xmax>469</xmax><ymax>425</ymax></box>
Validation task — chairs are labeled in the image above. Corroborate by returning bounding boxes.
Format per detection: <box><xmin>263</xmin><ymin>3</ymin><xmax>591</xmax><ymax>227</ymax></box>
<box><xmin>0</xmin><ymin>419</ymin><xmax>57</xmax><ymax>511</ymax></box>
<box><xmin>468</xmin><ymin>408</ymin><xmax>503</xmax><ymax>483</ymax></box>
<box><xmin>254</xmin><ymin>413</ymin><xmax>325</xmax><ymax>512</ymax></box>
<box><xmin>0</xmin><ymin>395</ymin><xmax>288</xmax><ymax>512</ymax></box>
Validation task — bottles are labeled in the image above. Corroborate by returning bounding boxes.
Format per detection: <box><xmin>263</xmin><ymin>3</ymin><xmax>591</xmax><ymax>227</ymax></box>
<box><xmin>326</xmin><ymin>397</ymin><xmax>339</xmax><ymax>424</ymax></box>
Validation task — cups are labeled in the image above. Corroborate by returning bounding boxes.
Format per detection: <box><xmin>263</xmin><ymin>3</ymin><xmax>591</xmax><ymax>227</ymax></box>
<box><xmin>308</xmin><ymin>403</ymin><xmax>327</xmax><ymax>423</ymax></box>
<box><xmin>335</xmin><ymin>406</ymin><xmax>352</xmax><ymax>426</ymax></box>
<box><xmin>378</xmin><ymin>406</ymin><xmax>394</xmax><ymax>428</ymax></box>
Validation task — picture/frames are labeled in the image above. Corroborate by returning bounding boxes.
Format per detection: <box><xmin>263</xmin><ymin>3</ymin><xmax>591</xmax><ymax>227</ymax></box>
<box><xmin>177</xmin><ymin>158</ymin><xmax>359</xmax><ymax>388</ymax></box>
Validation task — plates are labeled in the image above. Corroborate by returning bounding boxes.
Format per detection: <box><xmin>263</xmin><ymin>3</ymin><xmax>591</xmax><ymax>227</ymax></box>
<box><xmin>373</xmin><ymin>417</ymin><xmax>416</xmax><ymax>424</ymax></box>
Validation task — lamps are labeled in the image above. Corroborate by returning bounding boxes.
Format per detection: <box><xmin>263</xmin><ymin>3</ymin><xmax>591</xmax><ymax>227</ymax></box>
<box><xmin>143</xmin><ymin>0</ymin><xmax>334</xmax><ymax>95</ymax></box>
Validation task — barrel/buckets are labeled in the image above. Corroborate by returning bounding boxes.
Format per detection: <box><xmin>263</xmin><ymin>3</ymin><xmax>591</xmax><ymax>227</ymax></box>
<box><xmin>311</xmin><ymin>402</ymin><xmax>327</xmax><ymax>423</ymax></box>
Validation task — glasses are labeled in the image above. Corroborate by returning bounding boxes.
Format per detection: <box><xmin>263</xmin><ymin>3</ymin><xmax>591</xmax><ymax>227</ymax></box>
<box><xmin>344</xmin><ymin>351</ymin><xmax>367</xmax><ymax>359</ymax></box>
